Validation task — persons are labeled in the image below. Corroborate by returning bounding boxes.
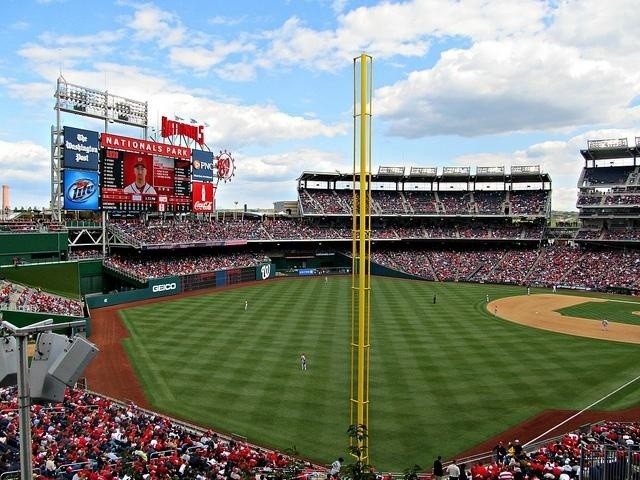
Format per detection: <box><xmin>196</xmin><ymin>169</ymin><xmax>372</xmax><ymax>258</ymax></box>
<box><xmin>486</xmin><ymin>293</ymin><xmax>491</xmax><ymax>303</ymax></box>
<box><xmin>124</xmin><ymin>151</ymin><xmax>157</xmax><ymax>194</ymax></box>
<box><xmin>299</xmin><ymin>351</ymin><xmax>308</xmax><ymax>375</ymax></box>
<box><xmin>493</xmin><ymin>305</ymin><xmax>498</xmax><ymax>317</ymax></box>
<box><xmin>432</xmin><ymin>292</ymin><xmax>438</xmax><ymax>304</ymax></box>
<box><xmin>601</xmin><ymin>315</ymin><xmax>609</xmax><ymax>332</ymax></box>
<box><xmin>1</xmin><ymin>206</ymin><xmax>307</xmax><ymax>318</ymax></box>
<box><xmin>300</xmin><ymin>184</ymin><xmax>640</xmax><ymax>295</ymax></box>
<box><xmin>0</xmin><ymin>380</ymin><xmax>640</xmax><ymax>480</ymax></box>
